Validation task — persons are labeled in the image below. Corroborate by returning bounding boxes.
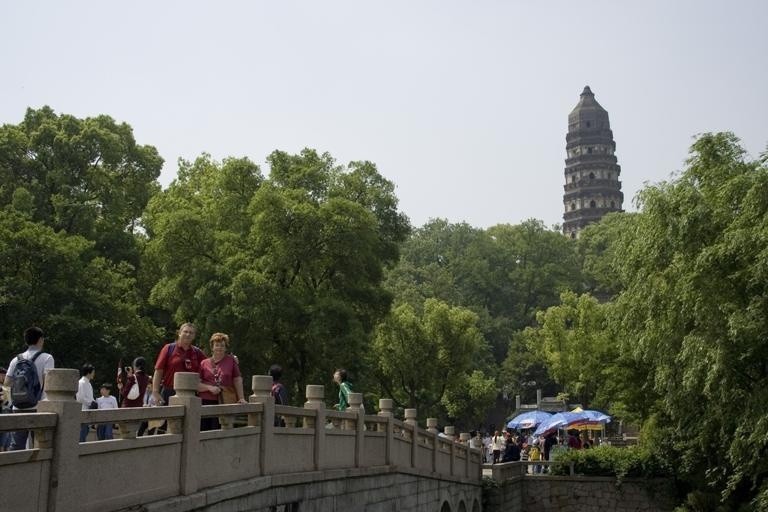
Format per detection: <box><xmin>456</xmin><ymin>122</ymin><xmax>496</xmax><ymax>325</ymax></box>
<box><xmin>4</xmin><ymin>325</ymin><xmax>56</xmax><ymax>451</ymax></box>
<box><xmin>77</xmin><ymin>318</ymin><xmax>289</xmax><ymax>442</ymax></box>
<box><xmin>333</xmin><ymin>369</ymin><xmax>355</xmax><ymax>411</ymax></box>
<box><xmin>458</xmin><ymin>428</ymin><xmax>609</xmax><ymax>475</ymax></box>
<box><xmin>0</xmin><ymin>367</ymin><xmax>8</xmax><ymax>446</ymax></box>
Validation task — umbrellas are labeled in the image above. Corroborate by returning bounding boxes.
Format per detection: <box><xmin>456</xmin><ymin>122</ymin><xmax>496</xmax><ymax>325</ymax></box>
<box><xmin>507</xmin><ymin>405</ymin><xmax>612</xmax><ymax>446</ymax></box>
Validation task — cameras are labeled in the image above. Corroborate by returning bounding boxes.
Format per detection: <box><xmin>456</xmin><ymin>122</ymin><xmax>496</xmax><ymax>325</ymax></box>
<box><xmin>125</xmin><ymin>366</ymin><xmax>130</xmax><ymax>371</ymax></box>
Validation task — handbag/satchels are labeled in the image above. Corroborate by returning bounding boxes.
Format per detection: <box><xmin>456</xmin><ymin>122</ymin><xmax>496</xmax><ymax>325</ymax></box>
<box><xmin>218</xmin><ymin>386</ymin><xmax>238</xmax><ymax>404</ymax></box>
<box><xmin>127</xmin><ymin>376</ymin><xmax>141</xmax><ymax>400</ymax></box>
<box><xmin>341</xmin><ymin>383</ymin><xmax>367</xmax><ymax>408</ymax></box>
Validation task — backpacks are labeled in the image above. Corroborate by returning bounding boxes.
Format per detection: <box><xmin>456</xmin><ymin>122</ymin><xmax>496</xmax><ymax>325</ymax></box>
<box><xmin>9</xmin><ymin>351</ymin><xmax>47</xmax><ymax>409</ymax></box>
